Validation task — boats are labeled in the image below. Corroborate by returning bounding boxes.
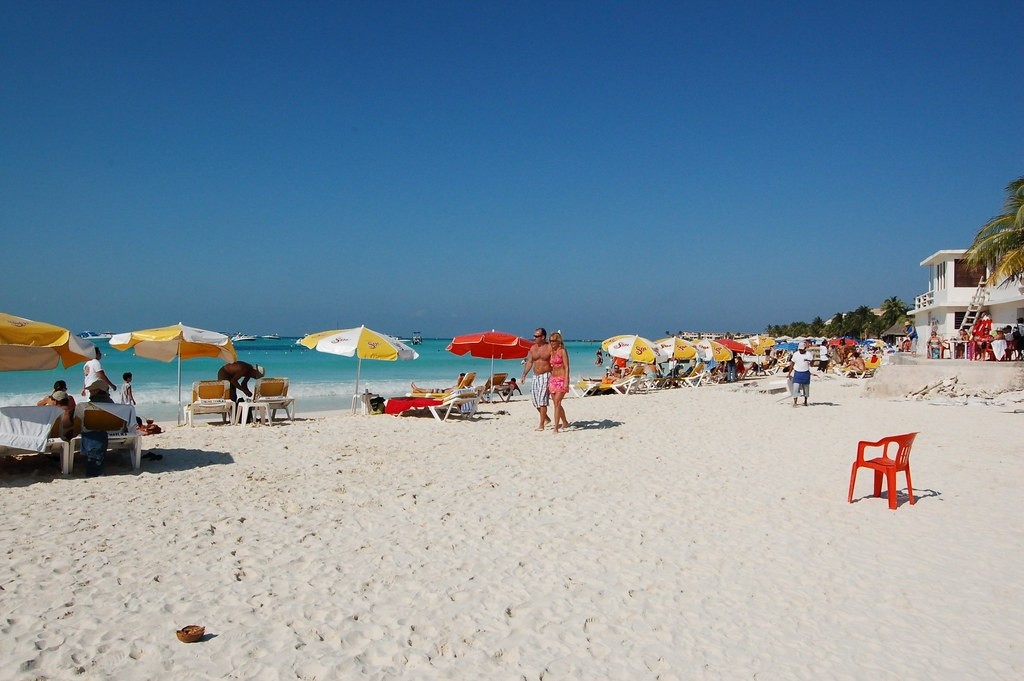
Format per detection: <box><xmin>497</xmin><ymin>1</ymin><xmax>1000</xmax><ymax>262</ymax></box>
<box><xmin>294</xmin><ymin>332</ymin><xmax>310</xmax><ymax>347</ymax></box>
<box><xmin>230</xmin><ymin>331</ymin><xmax>257</xmax><ymax>342</ymax></box>
<box><xmin>75</xmin><ymin>330</ymin><xmax>118</xmax><ymax>343</ymax></box>
<box><xmin>261</xmin><ymin>332</ymin><xmax>280</xmax><ymax>340</ymax></box>
<box><xmin>410</xmin><ymin>331</ymin><xmax>423</xmax><ymax>345</ymax></box>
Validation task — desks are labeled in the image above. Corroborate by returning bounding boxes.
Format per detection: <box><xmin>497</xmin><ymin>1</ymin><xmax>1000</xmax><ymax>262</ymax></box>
<box><xmin>945</xmin><ymin>340</ymin><xmax>970</xmax><ymax>360</ymax></box>
<box><xmin>350</xmin><ymin>393</ymin><xmax>379</xmax><ymax>415</ymax></box>
<box><xmin>235</xmin><ymin>401</ymin><xmax>274</xmax><ymax>429</ymax></box>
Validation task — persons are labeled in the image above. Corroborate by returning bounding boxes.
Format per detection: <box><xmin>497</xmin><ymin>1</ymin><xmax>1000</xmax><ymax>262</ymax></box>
<box><xmin>410</xmin><ymin>372</ymin><xmax>466</xmax><ymax>394</ymax></box>
<box><xmin>926</xmin><ymin>330</ymin><xmax>945</xmax><ymax>359</ymax></box>
<box><xmin>956</xmin><ymin>329</ymin><xmax>969</xmax><ymax>358</ymax></box>
<box><xmin>980</xmin><ymin>318</ymin><xmax>1024</xmax><ymax>361</ymax></box>
<box><xmin>119</xmin><ymin>371</ymin><xmax>136</xmax><ymax>406</ymax></box>
<box><xmin>493</xmin><ymin>377</ymin><xmax>523</xmax><ymax>395</ymax></box>
<box><xmin>217</xmin><ymin>361</ymin><xmax>264</xmax><ymax>424</ymax></box>
<box><xmin>903</xmin><ymin>321</ymin><xmax>919</xmax><ymax>356</ymax></box>
<box><xmin>521</xmin><ymin>328</ymin><xmax>571</xmax><ymax>433</ymax></box>
<box><xmin>38</xmin><ymin>380</ymin><xmax>116</xmax><ymax>440</ymax></box>
<box><xmin>596</xmin><ymin>341</ymin><xmax>883</xmax><ymax>408</ymax></box>
<box><xmin>81</xmin><ymin>346</ymin><xmax>118</xmax><ymax>404</ymax></box>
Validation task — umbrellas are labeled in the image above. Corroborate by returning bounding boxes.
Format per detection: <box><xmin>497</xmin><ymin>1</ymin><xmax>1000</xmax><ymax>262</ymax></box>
<box><xmin>109</xmin><ymin>322</ymin><xmax>237</xmax><ymax>424</ymax></box>
<box><xmin>445</xmin><ymin>328</ymin><xmax>535</xmax><ymax>403</ymax></box>
<box><xmin>603</xmin><ymin>334</ymin><xmax>889</xmax><ymax>379</ymax></box>
<box><xmin>300</xmin><ymin>325</ymin><xmax>420</xmax><ymax>414</ymax></box>
<box><xmin>0</xmin><ymin>313</ymin><xmax>97</xmax><ymax>372</ymax></box>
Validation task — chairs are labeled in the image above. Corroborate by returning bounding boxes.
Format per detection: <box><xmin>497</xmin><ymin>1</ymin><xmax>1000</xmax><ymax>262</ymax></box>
<box><xmin>570</xmin><ymin>343</ymin><xmax>886</xmax><ymax>399</ymax></box>
<box><xmin>68</xmin><ymin>401</ymin><xmax>143</xmax><ymax>477</ymax></box>
<box><xmin>383</xmin><ymin>373</ymin><xmax>515</xmax><ymax>423</ymax></box>
<box><xmin>0</xmin><ymin>405</ymin><xmax>69</xmax><ymax>475</ymax></box>
<box><xmin>926</xmin><ymin>335</ymin><xmax>1024</xmax><ymax>362</ymax></box>
<box><xmin>182</xmin><ymin>379</ymin><xmax>236</xmax><ymax>428</ymax></box>
<box><xmin>847</xmin><ymin>431</ymin><xmax>918</xmax><ymax>511</ymax></box>
<box><xmin>253</xmin><ymin>376</ymin><xmax>296</xmax><ymax>422</ymax></box>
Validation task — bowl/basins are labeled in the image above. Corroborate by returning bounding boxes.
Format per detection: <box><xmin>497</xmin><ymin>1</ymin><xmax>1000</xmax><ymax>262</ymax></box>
<box><xmin>175</xmin><ymin>631</ymin><xmax>204</xmax><ymax>642</ymax></box>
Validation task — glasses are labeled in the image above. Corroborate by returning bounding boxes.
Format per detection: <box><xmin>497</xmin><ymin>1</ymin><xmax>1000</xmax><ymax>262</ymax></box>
<box><xmin>549</xmin><ymin>339</ymin><xmax>558</xmax><ymax>343</ymax></box>
<box><xmin>532</xmin><ymin>334</ymin><xmax>544</xmax><ymax>338</ymax></box>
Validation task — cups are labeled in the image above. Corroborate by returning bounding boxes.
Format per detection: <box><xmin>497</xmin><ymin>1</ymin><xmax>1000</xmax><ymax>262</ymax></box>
<box><xmin>365</xmin><ymin>389</ymin><xmax>368</xmax><ymax>394</ymax></box>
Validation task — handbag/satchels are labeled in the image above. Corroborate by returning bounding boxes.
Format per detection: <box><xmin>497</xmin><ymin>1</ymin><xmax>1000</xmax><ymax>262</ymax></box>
<box><xmin>602</xmin><ymin>372</ymin><xmax>616</xmax><ymax>384</ymax></box>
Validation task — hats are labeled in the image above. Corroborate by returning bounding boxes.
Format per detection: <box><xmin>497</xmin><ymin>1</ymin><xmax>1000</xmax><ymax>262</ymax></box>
<box><xmin>53</xmin><ymin>391</ymin><xmax>69</xmax><ymax>401</ymax></box>
<box><xmin>255</xmin><ymin>364</ymin><xmax>265</xmax><ymax>380</ymax></box>
<box><xmin>84</xmin><ymin>379</ymin><xmax>110</xmax><ymax>394</ymax></box>
<box><xmin>797</xmin><ymin>343</ymin><xmax>807</xmax><ymax>350</ymax></box>
